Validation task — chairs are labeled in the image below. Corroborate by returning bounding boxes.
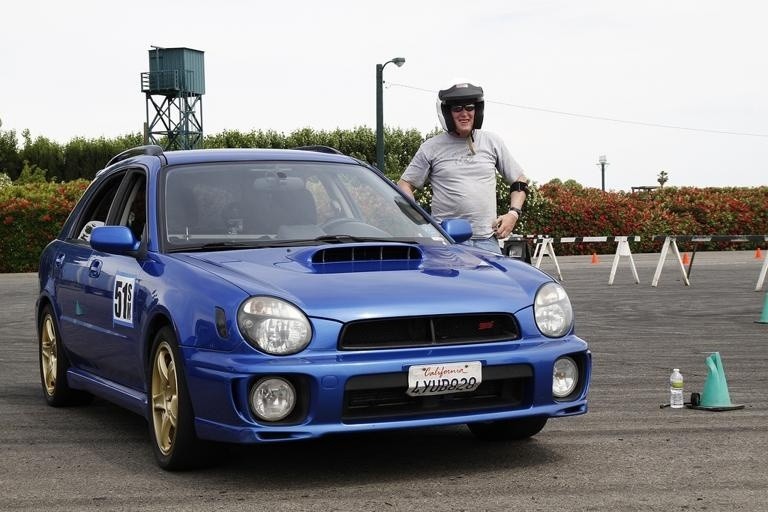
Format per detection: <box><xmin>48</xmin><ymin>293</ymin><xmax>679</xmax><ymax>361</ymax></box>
<box><xmin>262</xmin><ymin>188</ymin><xmax>326</xmax><ymax>240</ymax></box>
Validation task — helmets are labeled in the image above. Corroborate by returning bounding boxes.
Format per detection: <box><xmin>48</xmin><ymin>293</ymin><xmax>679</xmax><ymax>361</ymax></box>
<box><xmin>435</xmin><ymin>82</ymin><xmax>485</xmax><ymax>132</ymax></box>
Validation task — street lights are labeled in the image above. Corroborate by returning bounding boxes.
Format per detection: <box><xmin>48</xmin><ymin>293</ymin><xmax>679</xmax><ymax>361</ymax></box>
<box><xmin>597</xmin><ymin>155</ymin><xmax>610</xmax><ymax>192</ymax></box>
<box><xmin>375</xmin><ymin>58</ymin><xmax>406</xmax><ymax>175</ymax></box>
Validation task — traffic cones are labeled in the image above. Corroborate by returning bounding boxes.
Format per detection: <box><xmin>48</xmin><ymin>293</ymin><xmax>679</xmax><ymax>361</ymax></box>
<box><xmin>755</xmin><ymin>248</ymin><xmax>762</xmax><ymax>259</ymax></box>
<box><xmin>683</xmin><ymin>352</ymin><xmax>746</xmax><ymax>411</ymax></box>
<box><xmin>754</xmin><ymin>291</ymin><xmax>767</xmax><ymax>322</ymax></box>
<box><xmin>682</xmin><ymin>253</ymin><xmax>690</xmax><ymax>265</ymax></box>
<box><xmin>592</xmin><ymin>253</ymin><xmax>599</xmax><ymax>264</ymax></box>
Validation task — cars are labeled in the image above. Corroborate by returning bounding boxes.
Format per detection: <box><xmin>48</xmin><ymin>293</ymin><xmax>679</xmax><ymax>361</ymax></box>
<box><xmin>33</xmin><ymin>144</ymin><xmax>593</xmax><ymax>474</ymax></box>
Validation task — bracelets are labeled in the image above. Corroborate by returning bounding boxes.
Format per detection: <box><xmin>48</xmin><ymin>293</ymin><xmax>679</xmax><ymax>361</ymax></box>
<box><xmin>508</xmin><ymin>210</ymin><xmax>519</xmax><ymax>220</ymax></box>
<box><xmin>509</xmin><ymin>207</ymin><xmax>522</xmax><ymax>217</ymax></box>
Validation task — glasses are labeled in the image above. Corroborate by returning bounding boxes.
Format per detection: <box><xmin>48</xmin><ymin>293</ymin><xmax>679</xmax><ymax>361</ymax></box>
<box><xmin>450</xmin><ymin>104</ymin><xmax>476</xmax><ymax>112</ymax></box>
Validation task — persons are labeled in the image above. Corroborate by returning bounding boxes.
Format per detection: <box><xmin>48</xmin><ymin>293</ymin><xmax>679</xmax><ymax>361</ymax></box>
<box><xmin>398</xmin><ymin>83</ymin><xmax>528</xmax><ymax>256</ymax></box>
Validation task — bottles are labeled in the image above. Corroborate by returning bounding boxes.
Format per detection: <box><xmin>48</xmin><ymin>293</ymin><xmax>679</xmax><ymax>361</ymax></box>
<box><xmin>668</xmin><ymin>368</ymin><xmax>685</xmax><ymax>409</ymax></box>
